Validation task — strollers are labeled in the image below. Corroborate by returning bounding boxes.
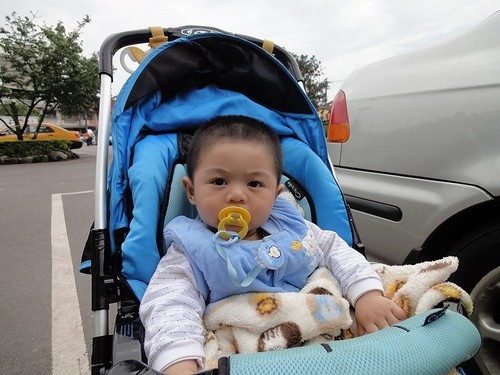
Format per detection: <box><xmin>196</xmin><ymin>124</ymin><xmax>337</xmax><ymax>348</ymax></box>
<box><xmin>80</xmin><ymin>24</ymin><xmax>490</xmax><ymax>374</ymax></box>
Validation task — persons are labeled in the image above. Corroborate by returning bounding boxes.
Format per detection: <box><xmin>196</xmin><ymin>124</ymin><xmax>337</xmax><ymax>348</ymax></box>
<box><xmin>138</xmin><ymin>114</ymin><xmax>409</xmax><ymax>375</ymax></box>
<box><xmin>84</xmin><ymin>127</ymin><xmax>97</xmax><ymax>146</ymax></box>
<box><xmin>30</xmin><ymin>131</ymin><xmax>38</xmax><ymax>140</ymax></box>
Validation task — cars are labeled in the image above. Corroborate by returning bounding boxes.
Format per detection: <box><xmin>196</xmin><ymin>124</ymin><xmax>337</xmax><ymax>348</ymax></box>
<box><xmin>0</xmin><ymin>123</ymin><xmax>83</xmax><ymax>151</ymax></box>
<box><xmin>61</xmin><ymin>126</ymin><xmax>95</xmax><ymax>149</ymax></box>
<box><xmin>327</xmin><ymin>10</ymin><xmax>499</xmax><ymax>373</ymax></box>
